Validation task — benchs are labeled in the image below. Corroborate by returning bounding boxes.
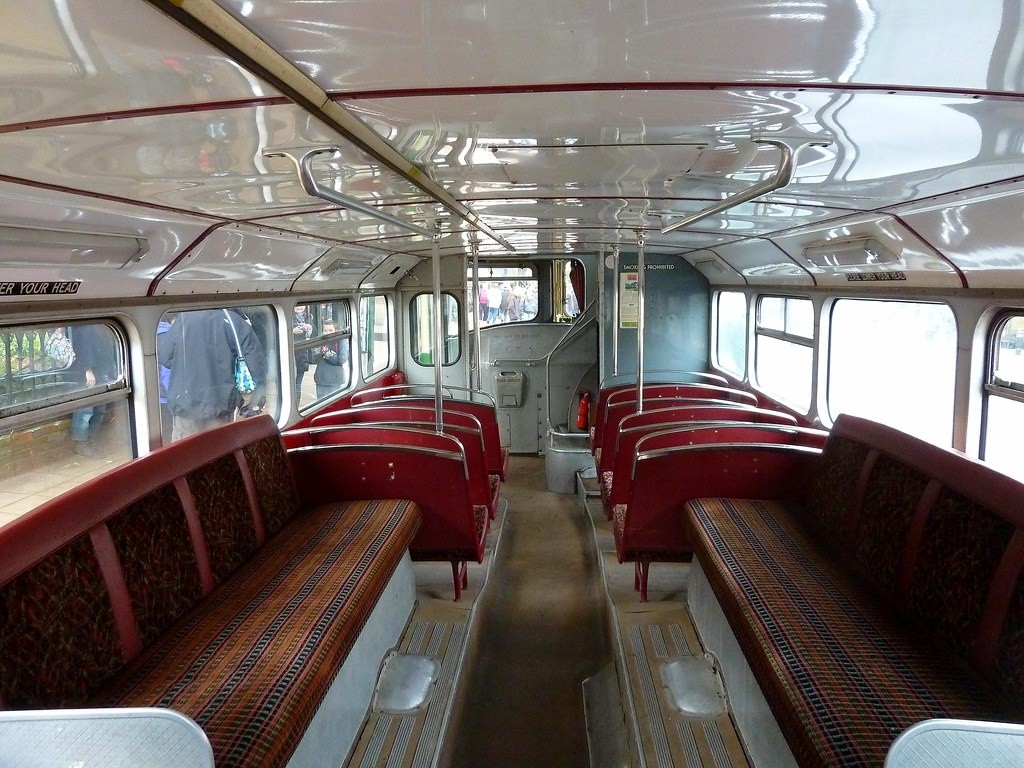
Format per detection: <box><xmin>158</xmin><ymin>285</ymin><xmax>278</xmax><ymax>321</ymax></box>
<box><xmin>1</xmin><ymin>384</ymin><xmax>510</xmax><ymax>767</ymax></box>
<box><xmin>589</xmin><ymin>368</ymin><xmax>1024</xmax><ymax>768</ymax></box>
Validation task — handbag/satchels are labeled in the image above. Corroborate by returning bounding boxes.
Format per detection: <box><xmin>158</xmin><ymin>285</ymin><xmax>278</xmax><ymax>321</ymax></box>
<box><xmin>234</xmin><ymin>354</ymin><xmax>257</xmax><ymax>395</ymax></box>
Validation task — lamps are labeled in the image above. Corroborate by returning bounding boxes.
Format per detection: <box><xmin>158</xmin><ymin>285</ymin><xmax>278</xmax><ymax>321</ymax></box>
<box><xmin>695</xmin><ymin>260</ymin><xmax>727</xmax><ymax>276</ymax></box>
<box><xmin>323</xmin><ymin>258</ymin><xmax>371</xmax><ymax>277</ymax></box>
<box><xmin>0</xmin><ymin>216</ymin><xmax>149</xmax><ymax>271</ymax></box>
<box><xmin>801</xmin><ymin>235</ymin><xmax>900</xmax><ymax>269</ymax></box>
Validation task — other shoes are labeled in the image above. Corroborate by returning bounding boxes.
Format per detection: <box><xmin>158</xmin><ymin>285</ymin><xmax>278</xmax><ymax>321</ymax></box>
<box><xmin>74</xmin><ymin>439</ymin><xmax>107</xmax><ymax>460</ymax></box>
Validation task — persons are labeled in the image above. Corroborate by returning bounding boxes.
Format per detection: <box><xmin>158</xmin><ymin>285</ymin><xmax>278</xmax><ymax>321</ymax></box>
<box><xmin>228</xmin><ymin>306</ymin><xmax>349</xmax><ymax>423</ymax></box>
<box><xmin>70</xmin><ymin>323</ymin><xmax>117</xmax><ymax>459</ymax></box>
<box><xmin>155</xmin><ymin>312</ymin><xmax>178</xmax><ymax>446</ymax></box>
<box><xmin>159</xmin><ymin>310</ymin><xmax>267</xmax><ymax>444</ymax></box>
<box><xmin>479</xmin><ymin>281</ymin><xmax>538</xmax><ymax>322</ymax></box>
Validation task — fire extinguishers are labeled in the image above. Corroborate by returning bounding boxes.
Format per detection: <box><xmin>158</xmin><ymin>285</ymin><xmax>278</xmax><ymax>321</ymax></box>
<box><xmin>392</xmin><ymin>371</ymin><xmax>405</xmax><ymax>396</ymax></box>
<box><xmin>575</xmin><ymin>388</ymin><xmax>593</xmax><ymax>429</ymax></box>
